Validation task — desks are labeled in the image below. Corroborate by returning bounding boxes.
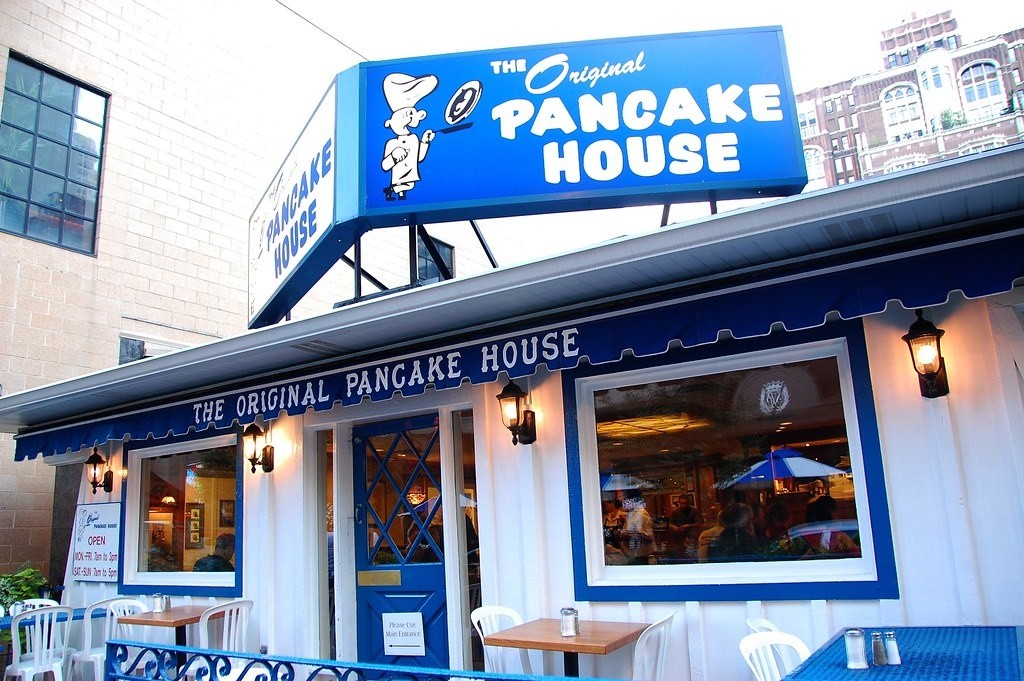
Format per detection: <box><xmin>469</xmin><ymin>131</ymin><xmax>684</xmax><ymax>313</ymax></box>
<box><xmin>485</xmin><ymin>618</ymin><xmax>652</xmax><ymax>678</ymax></box>
<box><xmin>0</xmin><ymin>607</ymin><xmax>134</xmax><ymax>681</ymax></box>
<box><xmin>781</xmin><ymin>625</ymin><xmax>1024</xmax><ymax>681</ymax></box>
<box><xmin>117</xmin><ymin>605</ymin><xmax>239</xmax><ymax>681</ymax></box>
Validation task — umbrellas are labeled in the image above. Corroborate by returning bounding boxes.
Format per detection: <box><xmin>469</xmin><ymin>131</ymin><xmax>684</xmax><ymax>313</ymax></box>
<box><xmin>714</xmin><ymin>449</ymin><xmax>847</xmax><ymax>490</ymax></box>
<box><xmin>600</xmin><ymin>473</ymin><xmax>656</xmax><ymax>491</ymax></box>
<box><xmin>397</xmin><ymin>492</ymin><xmax>476</xmax><ymax>517</ymax></box>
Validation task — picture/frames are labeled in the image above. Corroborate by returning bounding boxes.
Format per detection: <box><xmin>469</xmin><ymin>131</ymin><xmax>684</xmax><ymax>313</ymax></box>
<box><xmin>367</xmin><ymin>479</ymin><xmax>387</xmax><ymax>528</ymax></box>
<box><xmin>185</xmin><ymin>503</ymin><xmax>205</xmax><ymax>549</ymax></box>
<box><xmin>149</xmin><ymin>512</ymin><xmax>174</xmax><ymax>554</ymax></box>
<box><xmin>219</xmin><ymin>499</ymin><xmax>235</xmax><ymax>528</ymax></box>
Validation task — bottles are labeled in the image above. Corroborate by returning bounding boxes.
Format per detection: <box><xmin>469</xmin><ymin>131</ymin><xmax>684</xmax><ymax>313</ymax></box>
<box><xmin>165</xmin><ymin>595</ymin><xmax>172</xmax><ymax>612</ymax></box>
<box><xmin>152</xmin><ymin>593</ymin><xmax>164</xmax><ymax>613</ymax></box>
<box><xmin>870</xmin><ymin>631</ymin><xmax>887</xmax><ymax>667</ymax></box>
<box><xmin>561</xmin><ymin>607</ymin><xmax>580</xmax><ymax>637</ymax></box>
<box><xmin>14</xmin><ymin>600</ymin><xmax>24</xmax><ymax>616</ymax></box>
<box><xmin>844</xmin><ymin>627</ymin><xmax>870</xmax><ymax>670</ymax></box>
<box><xmin>884</xmin><ymin>631</ymin><xmax>902</xmax><ymax>665</ymax></box>
<box><xmin>26</xmin><ymin>603</ymin><xmax>32</xmax><ymax>620</ymax></box>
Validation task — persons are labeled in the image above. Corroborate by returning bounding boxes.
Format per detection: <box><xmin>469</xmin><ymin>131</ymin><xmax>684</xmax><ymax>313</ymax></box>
<box><xmin>602</xmin><ymin>489</ymin><xmax>657</xmax><ymax>564</ymax></box>
<box><xmin>795</xmin><ymin>495</ymin><xmax>860</xmax><ymax>557</ymax></box>
<box><xmin>193</xmin><ymin>533</ymin><xmax>235</xmax><ymax>572</ymax></box>
<box><xmin>400</xmin><ymin>521</ymin><xmax>442</xmax><ymax>562</ymax></box>
<box><xmin>707</xmin><ymin>503</ymin><xmax>794</xmax><ymax>557</ymax></box>
<box><xmin>669</xmin><ymin>495</ymin><xmax>705</xmax><ymax>550</ymax></box>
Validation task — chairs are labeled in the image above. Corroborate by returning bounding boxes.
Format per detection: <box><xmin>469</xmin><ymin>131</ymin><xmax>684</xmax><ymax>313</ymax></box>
<box><xmin>470</xmin><ymin>606</ymin><xmax>533</xmax><ymax>675</ymax></box>
<box><xmin>3</xmin><ymin>605</ymin><xmax>74</xmax><ymax>681</ymax></box>
<box><xmin>739</xmin><ymin>631</ymin><xmax>811</xmax><ymax>681</ymax></box>
<box><xmin>65</xmin><ymin>596</ymin><xmax>137</xmax><ymax>681</ymax></box>
<box><xmin>463</xmin><ymin>583</ymin><xmax>483</xmax><ymax>636</ymax></box>
<box><xmin>179</xmin><ymin>600</ymin><xmax>254</xmax><ymax>681</ymax></box>
<box><xmin>110</xmin><ymin>600</ymin><xmax>170</xmax><ymax>681</ymax></box>
<box><xmin>632</xmin><ymin>611</ymin><xmax>679</xmax><ymax>681</ymax></box>
<box><xmin>9</xmin><ymin>599</ymin><xmax>78</xmax><ymax>681</ymax></box>
<box><xmin>746</xmin><ymin>618</ymin><xmax>793</xmax><ymax>674</ymax></box>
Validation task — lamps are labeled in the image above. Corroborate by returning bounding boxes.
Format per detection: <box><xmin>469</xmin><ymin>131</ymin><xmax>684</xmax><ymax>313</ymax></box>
<box><xmin>84</xmin><ymin>447</ymin><xmax>113</xmax><ymax>495</ymax></box>
<box><xmin>240</xmin><ymin>423</ymin><xmax>274</xmax><ymax>474</ymax></box>
<box><xmin>902</xmin><ymin>308</ymin><xmax>950</xmax><ymax>398</ymax></box>
<box><xmin>495</xmin><ymin>380</ymin><xmax>537</xmax><ymax>446</ymax></box>
